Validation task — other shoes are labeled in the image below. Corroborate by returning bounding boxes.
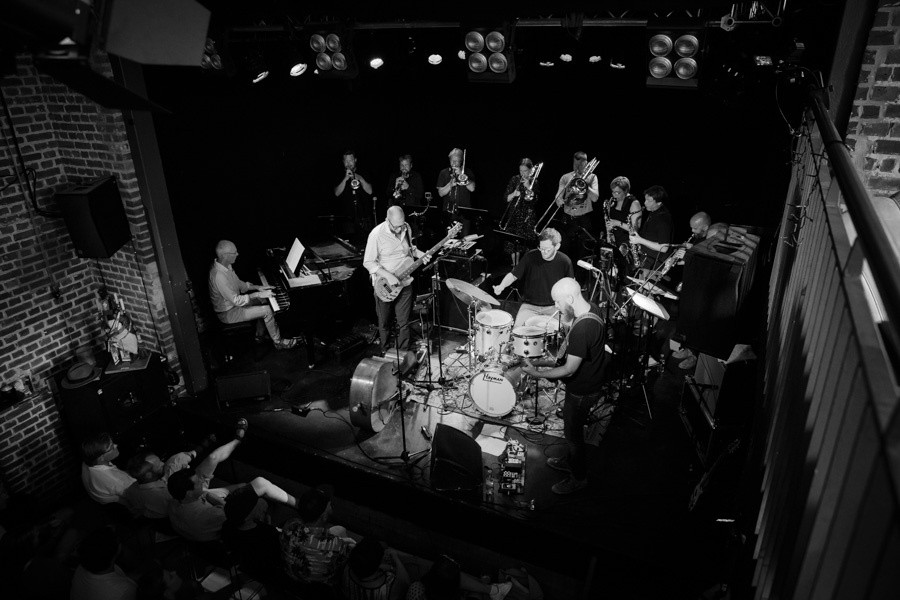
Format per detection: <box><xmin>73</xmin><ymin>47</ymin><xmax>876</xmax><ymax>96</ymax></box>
<box><xmin>547</xmin><ymin>457</ymin><xmax>574</xmax><ymax>474</ymax></box>
<box><xmin>274</xmin><ymin>337</ymin><xmax>297</xmax><ymax>350</ymax></box>
<box><xmin>552</xmin><ymin>475</ymin><xmax>587</xmax><ymax>494</ymax></box>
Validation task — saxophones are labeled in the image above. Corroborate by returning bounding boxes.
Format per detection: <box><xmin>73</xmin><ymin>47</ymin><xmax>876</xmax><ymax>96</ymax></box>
<box><xmin>603</xmin><ymin>196</ymin><xmax>616</xmax><ymax>243</ymax></box>
<box><xmin>627</xmin><ymin>207</ymin><xmax>646</xmax><ymax>267</ymax></box>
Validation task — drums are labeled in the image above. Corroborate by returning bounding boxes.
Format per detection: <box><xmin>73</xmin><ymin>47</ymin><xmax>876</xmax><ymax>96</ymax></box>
<box><xmin>511</xmin><ymin>325</ymin><xmax>548</xmax><ymax>358</ymax></box>
<box><xmin>473</xmin><ymin>308</ymin><xmax>514</xmax><ymax>359</ymax></box>
<box><xmin>469</xmin><ymin>354</ymin><xmax>531</xmax><ymax>417</ymax></box>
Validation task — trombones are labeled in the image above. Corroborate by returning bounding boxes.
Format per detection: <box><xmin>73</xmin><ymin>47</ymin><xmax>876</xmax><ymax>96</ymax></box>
<box><xmin>499</xmin><ymin>162</ymin><xmax>544</xmax><ymax>230</ymax></box>
<box><xmin>446</xmin><ymin>149</ymin><xmax>470</xmax><ymax>214</ymax></box>
<box><xmin>533</xmin><ymin>157</ymin><xmax>600</xmax><ymax>236</ymax></box>
<box><xmin>662</xmin><ymin>234</ymin><xmax>696</xmax><ymax>276</ymax></box>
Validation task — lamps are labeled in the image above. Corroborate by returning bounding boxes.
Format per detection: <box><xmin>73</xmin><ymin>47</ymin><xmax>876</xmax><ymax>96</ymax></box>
<box><xmin>249</xmin><ymin>47</ymin><xmax>271</xmax><ymax>88</ymax></box>
<box><xmin>637</xmin><ymin>25</ymin><xmax>704</xmax><ymax>93</ymax></box>
<box><xmin>200</xmin><ymin>34</ymin><xmax>243</xmax><ymax>71</ymax></box>
<box><xmin>459</xmin><ymin>20</ymin><xmax>518</xmax><ymax>86</ymax></box>
<box><xmin>582</xmin><ymin>34</ymin><xmax>604</xmax><ymax>65</ymax></box>
<box><xmin>606</xmin><ymin>42</ymin><xmax>631</xmax><ymax>73</ymax></box>
<box><xmin>276</xmin><ymin>40</ymin><xmax>309</xmax><ymax>81</ymax></box>
<box><xmin>532</xmin><ymin>32</ymin><xmax>555</xmax><ymax>67</ymax></box>
<box><xmin>308</xmin><ymin>26</ymin><xmax>372</xmax><ymax>70</ymax></box>
<box><xmin>28</xmin><ymin>0</ymin><xmax>96</xmax><ymax>65</ymax></box>
<box><xmin>557</xmin><ymin>40</ymin><xmax>577</xmax><ymax>63</ymax></box>
<box><xmin>412</xmin><ymin>31</ymin><xmax>447</xmax><ymax>66</ymax></box>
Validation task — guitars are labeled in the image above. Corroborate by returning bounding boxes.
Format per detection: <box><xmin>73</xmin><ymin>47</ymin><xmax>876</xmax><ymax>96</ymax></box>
<box><xmin>375</xmin><ymin>220</ymin><xmax>464</xmax><ymax>301</ymax></box>
<box><xmin>349</xmin><ymin>337</ymin><xmax>433</xmax><ymax>433</ymax></box>
<box><xmin>502</xmin><ymin>355</ymin><xmax>567</xmax><ymax>383</ymax></box>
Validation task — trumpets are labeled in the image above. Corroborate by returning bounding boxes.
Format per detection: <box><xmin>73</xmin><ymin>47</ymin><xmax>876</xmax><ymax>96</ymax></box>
<box><xmin>392</xmin><ymin>171</ymin><xmax>405</xmax><ymax>199</ymax></box>
<box><xmin>349</xmin><ymin>167</ymin><xmax>361</xmax><ymax>195</ymax></box>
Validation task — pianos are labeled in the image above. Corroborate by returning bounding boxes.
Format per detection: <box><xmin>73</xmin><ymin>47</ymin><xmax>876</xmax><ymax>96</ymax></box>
<box><xmin>255</xmin><ymin>231</ymin><xmax>363</xmax><ymax>330</ymax></box>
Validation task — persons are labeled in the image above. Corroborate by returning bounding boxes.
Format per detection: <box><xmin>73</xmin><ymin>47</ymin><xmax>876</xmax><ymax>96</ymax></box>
<box><xmin>0</xmin><ymin>418</ymin><xmax>512</xmax><ymax>600</ymax></box>
<box><xmin>603</xmin><ymin>175</ymin><xmax>727</xmax><ymax>371</ymax></box>
<box><xmin>362</xmin><ymin>206</ymin><xmax>432</xmax><ymax>355</ymax></box>
<box><xmin>385</xmin><ymin>156</ymin><xmax>422</xmax><ymax>222</ymax></box>
<box><xmin>210</xmin><ymin>241</ymin><xmax>295</xmax><ymax>349</ymax></box>
<box><xmin>494</xmin><ymin>227</ymin><xmax>575</xmax><ymax>329</ymax></box>
<box><xmin>436</xmin><ymin>148</ymin><xmax>475</xmax><ymax>243</ymax></box>
<box><xmin>554</xmin><ymin>152</ymin><xmax>599</xmax><ymax>257</ymax></box>
<box><xmin>522</xmin><ymin>277</ymin><xmax>605</xmax><ymax>490</ymax></box>
<box><xmin>332</xmin><ymin>149</ymin><xmax>372</xmax><ymax>239</ymax></box>
<box><xmin>504</xmin><ymin>158</ymin><xmax>538</xmax><ymax>267</ymax></box>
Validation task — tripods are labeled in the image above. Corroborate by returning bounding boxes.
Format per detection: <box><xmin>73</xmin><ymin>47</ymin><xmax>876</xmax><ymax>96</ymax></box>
<box><xmin>371</xmin><ymin>264</ymin><xmax>472</xmax><ymax>482</ymax></box>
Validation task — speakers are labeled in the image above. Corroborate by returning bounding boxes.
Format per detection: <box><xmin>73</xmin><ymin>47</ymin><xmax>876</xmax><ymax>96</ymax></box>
<box><xmin>106</xmin><ymin>0</ymin><xmax>211</xmax><ymax>65</ymax></box>
<box><xmin>430</xmin><ymin>423</ymin><xmax>483</xmax><ymax>491</ymax></box>
<box><xmin>54</xmin><ymin>175</ymin><xmax>133</xmax><ymax>258</ymax></box>
<box><xmin>670</xmin><ymin>231</ymin><xmax>766</xmax><ymax>420</ymax></box>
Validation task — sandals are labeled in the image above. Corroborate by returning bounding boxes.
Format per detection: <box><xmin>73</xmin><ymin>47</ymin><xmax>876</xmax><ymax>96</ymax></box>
<box><xmin>234</xmin><ymin>418</ymin><xmax>249</xmax><ymax>442</ymax></box>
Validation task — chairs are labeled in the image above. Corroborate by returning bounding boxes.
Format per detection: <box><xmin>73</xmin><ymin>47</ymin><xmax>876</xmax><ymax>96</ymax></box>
<box><xmin>93</xmin><ymin>500</ymin><xmax>227</xmax><ymax>582</ymax></box>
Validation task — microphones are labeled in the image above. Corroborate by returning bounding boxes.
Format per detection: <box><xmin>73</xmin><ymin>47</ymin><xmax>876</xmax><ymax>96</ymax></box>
<box><xmin>421</xmin><ymin>259</ymin><xmax>440</xmax><ymax>271</ymax></box>
<box><xmin>666</xmin><ymin>243</ymin><xmax>694</xmax><ymax>249</ymax></box>
<box><xmin>691</xmin><ymin>382</ymin><xmax>718</xmax><ymax>390</ymax></box>
<box><xmin>577</xmin><ymin>259</ymin><xmax>601</xmax><ymax>273</ymax></box>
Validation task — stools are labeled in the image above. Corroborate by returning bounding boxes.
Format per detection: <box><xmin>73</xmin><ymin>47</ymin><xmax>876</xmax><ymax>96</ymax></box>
<box><xmin>215</xmin><ymin>321</ymin><xmax>257</xmax><ymax>369</ymax></box>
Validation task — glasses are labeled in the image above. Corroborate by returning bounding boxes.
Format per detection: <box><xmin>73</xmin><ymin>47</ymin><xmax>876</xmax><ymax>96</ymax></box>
<box><xmin>388</xmin><ymin>220</ymin><xmax>406</xmax><ymax>229</ymax></box>
<box><xmin>536</xmin><ymin>245</ymin><xmax>552</xmax><ymax>253</ymax></box>
<box><xmin>611</xmin><ymin>191</ymin><xmax>622</xmax><ymax>196</ymax></box>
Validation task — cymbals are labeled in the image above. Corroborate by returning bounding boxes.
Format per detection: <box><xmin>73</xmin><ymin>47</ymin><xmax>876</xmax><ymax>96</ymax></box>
<box><xmin>445</xmin><ymin>279</ymin><xmax>501</xmax><ymax>311</ymax></box>
